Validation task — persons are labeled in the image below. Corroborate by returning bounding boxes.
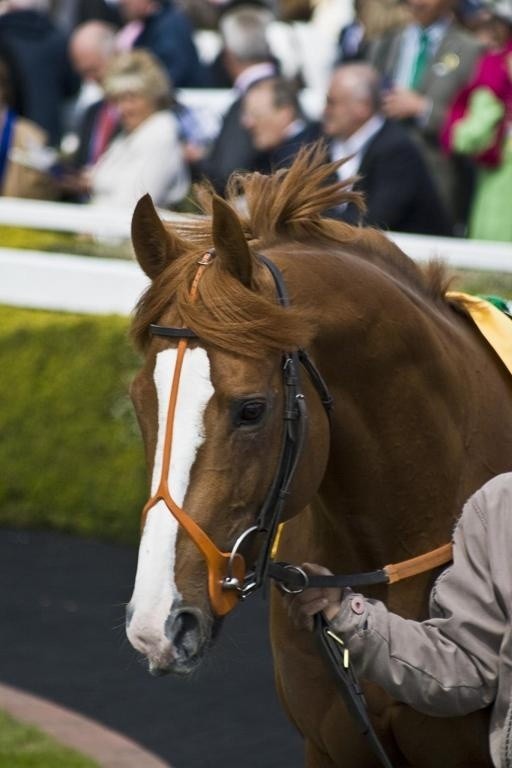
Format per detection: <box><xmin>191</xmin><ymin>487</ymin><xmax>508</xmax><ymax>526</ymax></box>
<box><xmin>283</xmin><ymin>473</ymin><xmax>512</xmax><ymax>767</ymax></box>
<box><xmin>1</xmin><ymin>0</ymin><xmax>512</xmax><ymax>238</ymax></box>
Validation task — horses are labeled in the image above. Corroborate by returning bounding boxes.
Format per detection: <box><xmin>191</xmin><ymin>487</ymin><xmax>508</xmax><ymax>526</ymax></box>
<box><xmin>121</xmin><ymin>136</ymin><xmax>512</xmax><ymax>768</ymax></box>
